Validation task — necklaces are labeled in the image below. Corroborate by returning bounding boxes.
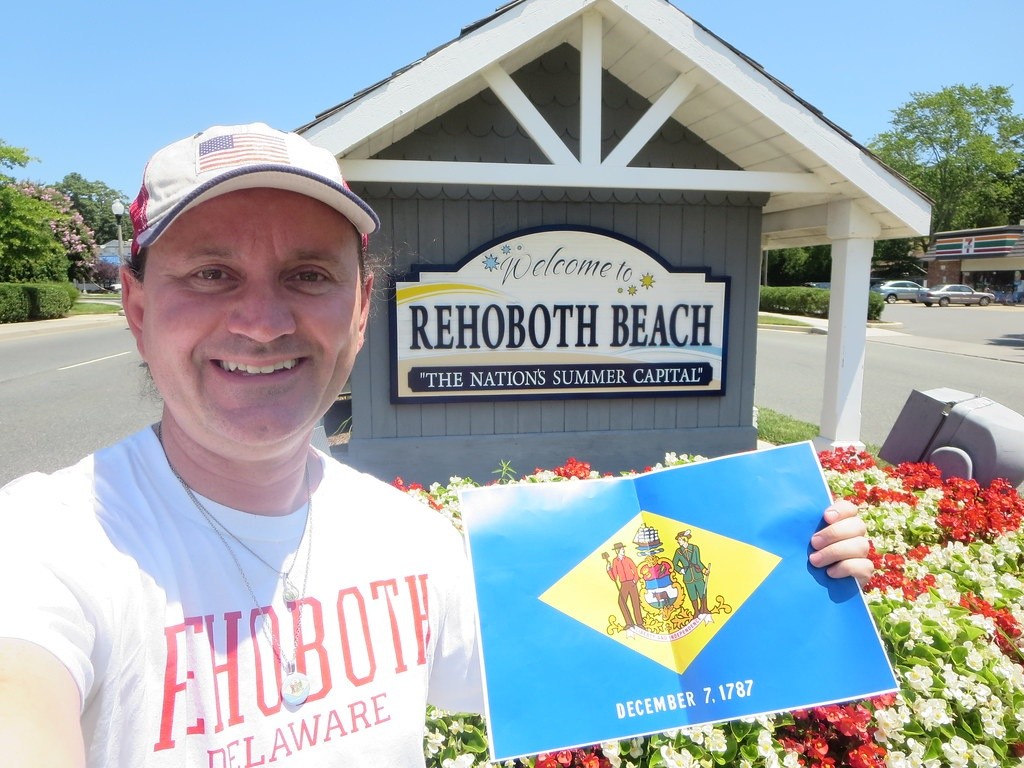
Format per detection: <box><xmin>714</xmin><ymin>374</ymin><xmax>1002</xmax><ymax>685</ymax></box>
<box><xmin>157</xmin><ymin>422</ymin><xmax>312</xmax><ymax>707</ymax></box>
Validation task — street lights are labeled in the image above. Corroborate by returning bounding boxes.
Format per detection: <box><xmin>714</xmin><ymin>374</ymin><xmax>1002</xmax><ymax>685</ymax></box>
<box><xmin>111</xmin><ymin>198</ymin><xmax>126</xmax><ymax>264</ymax></box>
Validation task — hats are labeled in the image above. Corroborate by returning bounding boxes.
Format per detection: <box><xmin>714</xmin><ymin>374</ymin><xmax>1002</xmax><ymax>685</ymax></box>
<box><xmin>129</xmin><ymin>122</ymin><xmax>380</xmax><ymax>258</ymax></box>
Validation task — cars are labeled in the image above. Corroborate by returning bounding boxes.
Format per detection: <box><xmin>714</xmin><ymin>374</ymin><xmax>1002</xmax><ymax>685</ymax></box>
<box><xmin>872</xmin><ymin>280</ymin><xmax>928</xmax><ymax>304</ymax></box>
<box><xmin>919</xmin><ymin>285</ymin><xmax>996</xmax><ymax>308</ymax></box>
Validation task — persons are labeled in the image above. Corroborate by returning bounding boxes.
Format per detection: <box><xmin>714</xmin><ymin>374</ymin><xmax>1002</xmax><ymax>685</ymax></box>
<box><xmin>0</xmin><ymin>123</ymin><xmax>873</xmax><ymax>768</ymax></box>
